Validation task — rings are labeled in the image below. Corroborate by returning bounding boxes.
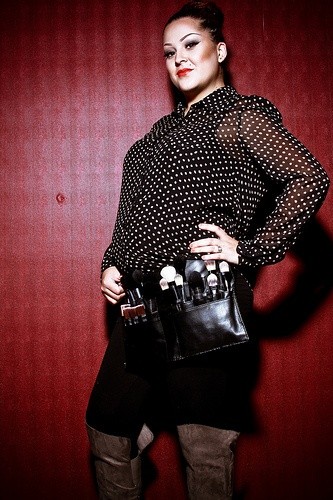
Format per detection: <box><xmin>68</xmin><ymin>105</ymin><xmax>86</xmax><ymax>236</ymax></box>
<box><xmin>217</xmin><ymin>253</ymin><xmax>220</xmax><ymax>260</ymax></box>
<box><xmin>216</xmin><ymin>245</ymin><xmax>222</xmax><ymax>253</ymax></box>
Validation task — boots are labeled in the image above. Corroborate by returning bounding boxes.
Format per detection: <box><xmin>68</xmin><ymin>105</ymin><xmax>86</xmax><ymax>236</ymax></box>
<box><xmin>85</xmin><ymin>421</ymin><xmax>154</xmax><ymax>500</ymax></box>
<box><xmin>176</xmin><ymin>424</ymin><xmax>239</xmax><ymax>500</ymax></box>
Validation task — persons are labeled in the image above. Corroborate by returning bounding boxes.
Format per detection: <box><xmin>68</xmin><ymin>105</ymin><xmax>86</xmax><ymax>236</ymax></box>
<box><xmin>86</xmin><ymin>0</ymin><xmax>330</xmax><ymax>500</ymax></box>
